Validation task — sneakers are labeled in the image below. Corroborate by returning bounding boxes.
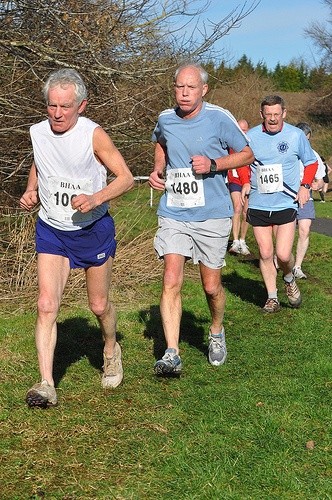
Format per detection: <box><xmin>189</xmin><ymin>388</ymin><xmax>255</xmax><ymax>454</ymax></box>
<box><xmin>264</xmin><ymin>298</ymin><xmax>280</xmax><ymax>313</ymax></box>
<box><xmin>283</xmin><ymin>274</ymin><xmax>302</xmax><ymax>308</ymax></box>
<box><xmin>101</xmin><ymin>341</ymin><xmax>124</xmax><ymax>389</ymax></box>
<box><xmin>208</xmin><ymin>325</ymin><xmax>227</xmax><ymax>366</ymax></box>
<box><xmin>240</xmin><ymin>241</ymin><xmax>251</xmax><ymax>256</ymax></box>
<box><xmin>155</xmin><ymin>348</ymin><xmax>181</xmax><ymax>377</ymax></box>
<box><xmin>229</xmin><ymin>242</ymin><xmax>241</xmax><ymax>254</ymax></box>
<box><xmin>26</xmin><ymin>379</ymin><xmax>57</xmax><ymax>407</ymax></box>
<box><xmin>294</xmin><ymin>267</ymin><xmax>306</xmax><ymax>279</ymax></box>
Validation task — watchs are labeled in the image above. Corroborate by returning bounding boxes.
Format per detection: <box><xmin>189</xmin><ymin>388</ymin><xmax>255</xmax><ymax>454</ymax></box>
<box><xmin>301</xmin><ymin>183</ymin><xmax>310</xmax><ymax>189</ymax></box>
<box><xmin>208</xmin><ymin>159</ymin><xmax>217</xmax><ymax>178</ymax></box>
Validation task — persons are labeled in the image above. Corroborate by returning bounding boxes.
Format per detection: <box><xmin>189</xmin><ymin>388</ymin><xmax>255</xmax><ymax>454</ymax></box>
<box><xmin>228</xmin><ymin>96</ymin><xmax>329</xmax><ymax>310</ymax></box>
<box><xmin>19</xmin><ymin>68</ymin><xmax>135</xmax><ymax>406</ymax></box>
<box><xmin>149</xmin><ymin>63</ymin><xmax>255</xmax><ymax>374</ymax></box>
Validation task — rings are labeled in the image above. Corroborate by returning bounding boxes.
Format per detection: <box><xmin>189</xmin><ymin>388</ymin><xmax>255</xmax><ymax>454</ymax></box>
<box><xmin>80</xmin><ymin>205</ymin><xmax>82</xmax><ymax>210</ymax></box>
